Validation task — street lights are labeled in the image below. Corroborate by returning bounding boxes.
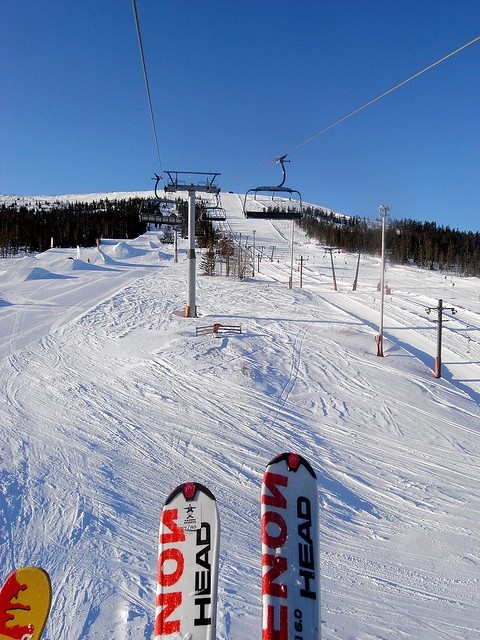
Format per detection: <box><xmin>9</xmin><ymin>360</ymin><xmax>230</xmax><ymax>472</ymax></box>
<box><xmin>425</xmin><ymin>299</ymin><xmax>457</xmax><ymax>378</ymax></box>
<box><xmin>252</xmin><ymin>229</ymin><xmax>256</xmax><ymax>276</ymax></box>
<box><xmin>325</xmin><ymin>248</ymin><xmax>342</xmax><ymax>291</ymax></box>
<box><xmin>378</xmin><ymin>204</ymin><xmax>390</xmax><ymax>358</ymax></box>
<box><xmin>295</xmin><ymin>256</ymin><xmax>309</xmax><ymax>288</ymax></box>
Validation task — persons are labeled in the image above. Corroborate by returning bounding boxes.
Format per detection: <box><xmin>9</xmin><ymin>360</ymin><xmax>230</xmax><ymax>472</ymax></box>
<box><xmin>154</xmin><ymin>209</ymin><xmax>163</xmax><ymax>229</ymax></box>
<box><xmin>167</xmin><ymin>209</ymin><xmax>177</xmax><ymax>230</ymax></box>
<box><xmin>161</xmin><ymin>238</ymin><xmax>174</xmax><ymax>244</ymax></box>
<box><xmin>262</xmin><ymin>206</ymin><xmax>302</xmax><ymax>220</ymax></box>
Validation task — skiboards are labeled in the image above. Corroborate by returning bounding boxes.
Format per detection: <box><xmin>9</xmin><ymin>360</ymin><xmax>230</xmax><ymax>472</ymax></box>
<box><xmin>0</xmin><ymin>567</ymin><xmax>53</xmax><ymax>640</ymax></box>
<box><xmin>155</xmin><ymin>452</ymin><xmax>320</xmax><ymax>640</ymax></box>
